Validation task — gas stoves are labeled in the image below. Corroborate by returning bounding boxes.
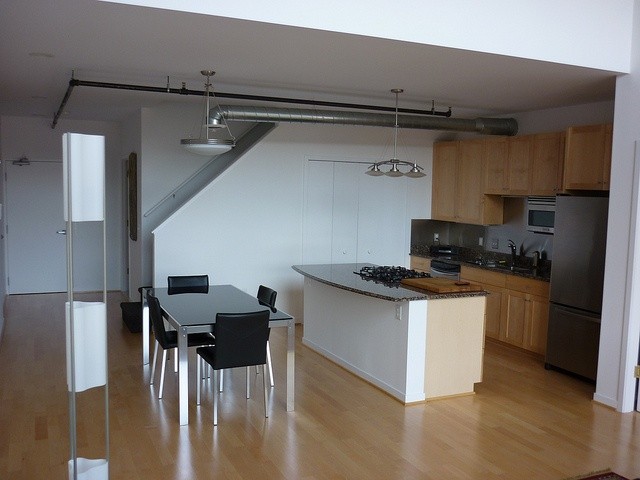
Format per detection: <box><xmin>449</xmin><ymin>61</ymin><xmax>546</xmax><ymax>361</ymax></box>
<box><xmin>352</xmin><ymin>266</ymin><xmax>432</xmax><ymax>283</ymax></box>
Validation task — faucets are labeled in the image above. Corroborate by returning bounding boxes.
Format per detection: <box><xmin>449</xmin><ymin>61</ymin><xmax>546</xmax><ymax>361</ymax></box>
<box><xmin>507</xmin><ymin>239</ymin><xmax>517</xmax><ymax>263</ymax></box>
<box><xmin>532</xmin><ymin>250</ymin><xmax>540</xmax><ymax>268</ymax></box>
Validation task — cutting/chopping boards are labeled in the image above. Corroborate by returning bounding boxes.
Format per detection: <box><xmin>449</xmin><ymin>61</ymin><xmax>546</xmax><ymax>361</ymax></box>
<box><xmin>400</xmin><ymin>276</ymin><xmax>483</xmax><ymax>293</ymax></box>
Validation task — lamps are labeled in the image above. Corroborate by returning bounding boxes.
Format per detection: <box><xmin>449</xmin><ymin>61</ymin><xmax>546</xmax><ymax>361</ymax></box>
<box><xmin>181</xmin><ymin>70</ymin><xmax>236</xmax><ymax>156</ymax></box>
<box><xmin>365</xmin><ymin>89</ymin><xmax>428</xmax><ymax>178</ymax></box>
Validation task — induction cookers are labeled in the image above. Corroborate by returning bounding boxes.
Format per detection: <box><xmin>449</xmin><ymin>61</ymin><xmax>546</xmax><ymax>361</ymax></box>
<box><xmin>432</xmin><ymin>254</ymin><xmax>468</xmax><ymax>265</ymax></box>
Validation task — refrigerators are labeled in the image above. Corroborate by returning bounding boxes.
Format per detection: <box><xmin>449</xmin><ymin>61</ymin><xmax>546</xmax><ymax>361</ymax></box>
<box><xmin>544</xmin><ymin>193</ymin><xmax>609</xmax><ymax>381</ymax></box>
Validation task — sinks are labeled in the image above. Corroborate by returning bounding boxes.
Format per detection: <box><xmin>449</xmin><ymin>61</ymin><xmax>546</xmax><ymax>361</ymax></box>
<box><xmin>484</xmin><ymin>261</ymin><xmax>528</xmax><ymax>272</ymax></box>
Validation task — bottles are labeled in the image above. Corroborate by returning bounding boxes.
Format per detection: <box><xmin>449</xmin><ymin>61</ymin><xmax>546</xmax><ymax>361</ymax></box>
<box><xmin>531</xmin><ymin>250</ymin><xmax>540</xmax><ymax>267</ymax></box>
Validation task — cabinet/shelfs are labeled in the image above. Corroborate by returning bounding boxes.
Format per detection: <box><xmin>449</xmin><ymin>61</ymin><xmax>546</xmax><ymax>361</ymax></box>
<box><xmin>485</xmin><ymin>133</ymin><xmax>574</xmax><ymax>195</ymax></box>
<box><xmin>459</xmin><ymin>262</ymin><xmax>502</xmax><ymax>342</ymax></box>
<box><xmin>500</xmin><ymin>268</ymin><xmax>550</xmax><ymax>358</ymax></box>
<box><xmin>432</xmin><ymin>140</ymin><xmax>504</xmax><ymax>225</ymax></box>
<box><xmin>564</xmin><ymin>125</ymin><xmax>613</xmax><ymax>191</ymax></box>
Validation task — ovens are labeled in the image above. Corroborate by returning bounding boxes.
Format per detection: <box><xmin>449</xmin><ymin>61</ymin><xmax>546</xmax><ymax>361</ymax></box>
<box><xmin>430</xmin><ymin>267</ymin><xmax>459</xmax><ymax>282</ymax></box>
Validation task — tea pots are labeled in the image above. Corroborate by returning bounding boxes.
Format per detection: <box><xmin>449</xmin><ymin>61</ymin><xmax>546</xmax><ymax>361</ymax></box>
<box><xmin>472</xmin><ymin>254</ymin><xmax>488</xmax><ymax>264</ymax></box>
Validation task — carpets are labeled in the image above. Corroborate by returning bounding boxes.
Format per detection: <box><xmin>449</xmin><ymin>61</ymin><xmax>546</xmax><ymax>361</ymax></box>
<box><xmin>566</xmin><ymin>467</ymin><xmax>630</xmax><ymax>480</ymax></box>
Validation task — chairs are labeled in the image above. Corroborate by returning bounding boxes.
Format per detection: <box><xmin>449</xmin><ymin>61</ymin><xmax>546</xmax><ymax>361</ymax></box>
<box><xmin>167</xmin><ymin>275</ymin><xmax>228</xmax><ymax>392</ymax></box>
<box><xmin>196</xmin><ymin>309</ymin><xmax>269</xmax><ymax>426</ymax></box>
<box><xmin>207</xmin><ymin>285</ymin><xmax>278</xmax><ymax>394</ymax></box>
<box><xmin>146</xmin><ymin>290</ymin><xmax>224</xmax><ymax>399</ymax></box>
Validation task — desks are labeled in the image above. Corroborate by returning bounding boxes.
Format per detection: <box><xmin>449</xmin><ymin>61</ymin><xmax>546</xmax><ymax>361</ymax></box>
<box><xmin>142</xmin><ymin>284</ymin><xmax>295</xmax><ymax>427</ymax></box>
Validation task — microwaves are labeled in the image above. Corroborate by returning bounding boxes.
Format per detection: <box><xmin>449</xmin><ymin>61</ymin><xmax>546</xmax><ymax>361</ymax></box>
<box><xmin>526</xmin><ymin>200</ymin><xmax>555</xmax><ymax>233</ymax></box>
